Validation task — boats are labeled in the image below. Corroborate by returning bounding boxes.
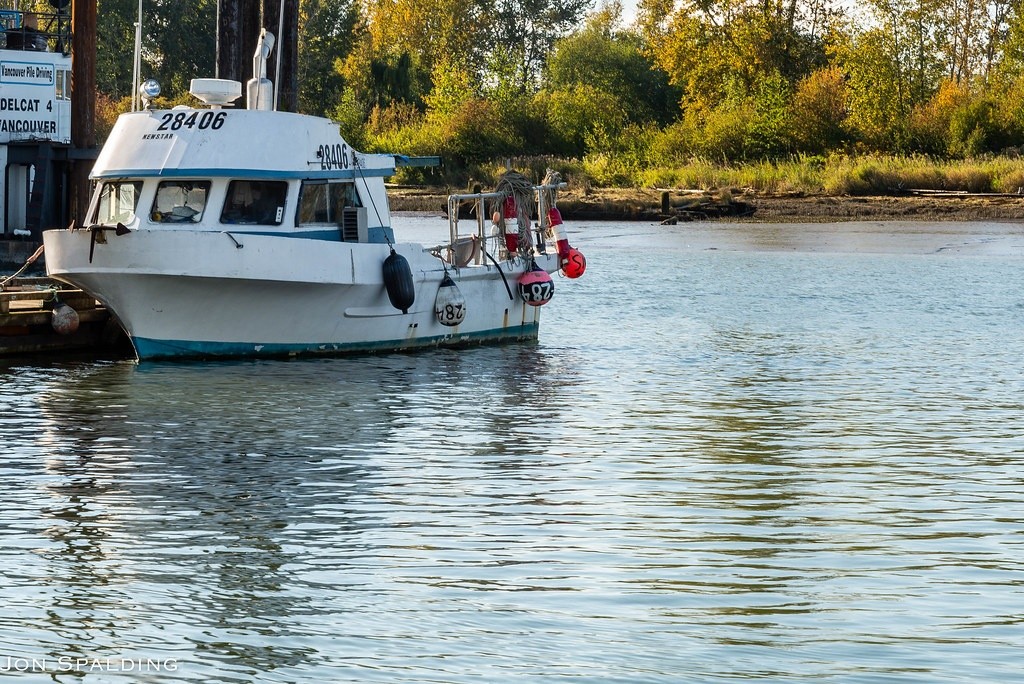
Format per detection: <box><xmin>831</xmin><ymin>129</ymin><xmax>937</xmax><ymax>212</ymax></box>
<box><xmin>42</xmin><ymin>0</ymin><xmax>564</xmax><ymax>364</ymax></box>
